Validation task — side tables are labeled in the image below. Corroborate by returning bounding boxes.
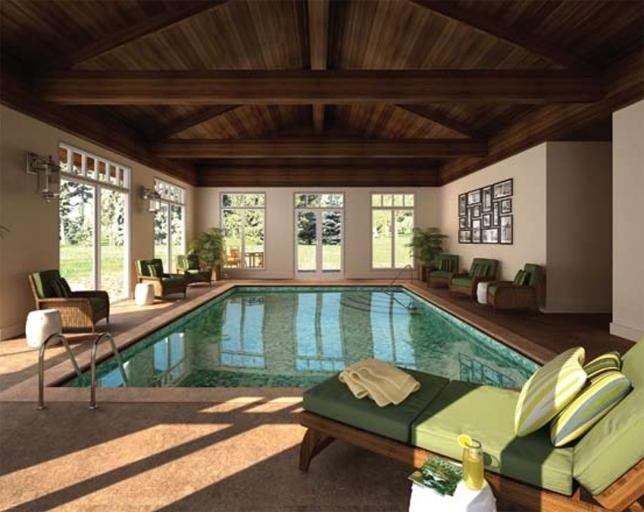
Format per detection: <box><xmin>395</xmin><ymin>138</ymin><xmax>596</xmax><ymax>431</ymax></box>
<box><xmin>408</xmin><ymin>475</ymin><xmax>497</xmax><ymax>512</ymax></box>
<box><xmin>134</xmin><ymin>283</ymin><xmax>154</xmax><ymax>306</ymax></box>
<box><xmin>25</xmin><ymin>309</ymin><xmax>63</xmax><ymax>348</ymax></box>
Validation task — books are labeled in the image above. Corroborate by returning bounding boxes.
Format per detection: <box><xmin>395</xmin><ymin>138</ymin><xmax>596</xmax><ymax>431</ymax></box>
<box><xmin>407</xmin><ymin>455</ymin><xmax>464</xmax><ymax>497</ymax></box>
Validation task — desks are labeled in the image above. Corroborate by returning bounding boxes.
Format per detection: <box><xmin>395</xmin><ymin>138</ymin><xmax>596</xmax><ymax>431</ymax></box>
<box><xmin>245</xmin><ymin>251</ymin><xmax>263</xmax><ymax>267</ymax></box>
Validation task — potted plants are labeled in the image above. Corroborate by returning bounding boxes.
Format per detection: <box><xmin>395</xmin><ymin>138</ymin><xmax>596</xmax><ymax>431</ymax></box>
<box><xmin>404</xmin><ymin>227</ymin><xmax>448</xmax><ymax>281</ymax></box>
<box><xmin>189</xmin><ymin>228</ymin><xmax>223</xmax><ymax>280</ymax></box>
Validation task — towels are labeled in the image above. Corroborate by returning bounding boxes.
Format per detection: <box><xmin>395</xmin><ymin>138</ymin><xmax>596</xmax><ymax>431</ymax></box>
<box><xmin>338</xmin><ymin>357</ymin><xmax>421</xmax><ymax>408</ymax></box>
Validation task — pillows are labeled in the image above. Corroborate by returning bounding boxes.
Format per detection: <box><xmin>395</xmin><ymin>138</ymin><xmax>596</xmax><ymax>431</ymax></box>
<box><xmin>147</xmin><ymin>263</ymin><xmax>163</xmax><ymax>277</ymax></box>
<box><xmin>514</xmin><ymin>346</ymin><xmax>588</xmax><ymax>436</ymax></box>
<box><xmin>183</xmin><ymin>258</ymin><xmax>198</xmax><ymax>270</ymax></box>
<box><xmin>550</xmin><ymin>350</ymin><xmax>632</xmax><ymax>447</ymax></box>
<box><xmin>50</xmin><ymin>277</ymin><xmax>73</xmax><ymax>297</ymax></box>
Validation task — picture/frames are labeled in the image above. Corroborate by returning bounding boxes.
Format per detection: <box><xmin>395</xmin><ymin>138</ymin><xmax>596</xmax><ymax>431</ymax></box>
<box><xmin>459</xmin><ymin>177</ymin><xmax>513</xmax><ymax>245</ymax></box>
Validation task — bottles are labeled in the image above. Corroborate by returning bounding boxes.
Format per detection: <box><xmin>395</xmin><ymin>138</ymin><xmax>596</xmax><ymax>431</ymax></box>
<box><xmin>462</xmin><ymin>439</ymin><xmax>484</xmax><ymax>489</ymax></box>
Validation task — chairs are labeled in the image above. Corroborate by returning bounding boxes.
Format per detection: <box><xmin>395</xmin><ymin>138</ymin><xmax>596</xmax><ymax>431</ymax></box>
<box><xmin>134</xmin><ymin>259</ymin><xmax>187</xmax><ymax>301</ymax></box>
<box><xmin>140</xmin><ymin>185</ymin><xmax>161</xmax><ymax>214</ymax></box>
<box><xmin>224</xmin><ymin>245</ymin><xmax>241</xmax><ymax>268</ymax></box>
<box><xmin>176</xmin><ymin>255</ymin><xmax>212</xmax><ymax>288</ymax></box>
<box><xmin>296</xmin><ymin>339</ymin><xmax>644</xmax><ymax>512</ymax></box>
<box><xmin>28</xmin><ymin>270</ymin><xmax>109</xmax><ymax>333</ymax></box>
<box><xmin>426</xmin><ymin>255</ymin><xmax>542</xmax><ymax>316</ymax></box>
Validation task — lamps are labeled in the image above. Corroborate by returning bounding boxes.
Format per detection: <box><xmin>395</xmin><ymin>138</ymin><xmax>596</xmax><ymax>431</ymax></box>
<box><xmin>25</xmin><ymin>150</ymin><xmax>61</xmax><ymax>204</ymax></box>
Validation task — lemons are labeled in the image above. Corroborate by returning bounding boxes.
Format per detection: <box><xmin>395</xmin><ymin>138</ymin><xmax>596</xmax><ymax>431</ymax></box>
<box><xmin>457</xmin><ymin>435</ymin><xmax>472</xmax><ymax>448</ymax></box>
